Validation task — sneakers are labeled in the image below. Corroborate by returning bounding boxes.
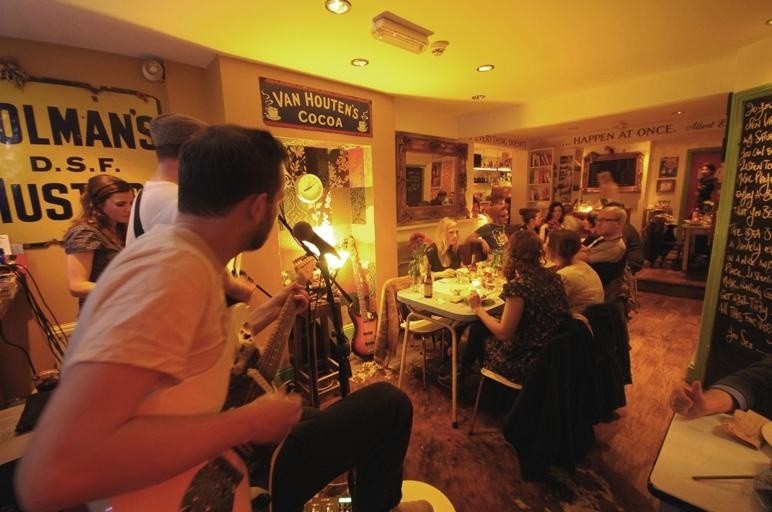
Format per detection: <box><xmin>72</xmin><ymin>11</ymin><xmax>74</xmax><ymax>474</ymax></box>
<box><xmin>389</xmin><ymin>500</ymin><xmax>434</xmax><ymax>512</ymax></box>
<box><xmin>438</xmin><ymin>372</ymin><xmax>462</xmax><ymax>391</ymax></box>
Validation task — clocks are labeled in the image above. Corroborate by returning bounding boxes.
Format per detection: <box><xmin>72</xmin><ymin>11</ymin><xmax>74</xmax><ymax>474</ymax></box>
<box><xmin>295</xmin><ymin>173</ymin><xmax>324</xmax><ymax>204</ymax></box>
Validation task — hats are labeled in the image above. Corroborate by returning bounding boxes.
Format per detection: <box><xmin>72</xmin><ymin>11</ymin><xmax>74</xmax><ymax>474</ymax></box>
<box><xmin>151</xmin><ymin>113</ymin><xmax>210</xmax><ymax>146</ymax></box>
<box><xmin>486</xmin><ymin>205</ymin><xmax>507</xmax><ymax>222</ymax></box>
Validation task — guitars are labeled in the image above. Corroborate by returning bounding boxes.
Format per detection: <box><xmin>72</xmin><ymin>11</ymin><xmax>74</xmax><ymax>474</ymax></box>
<box><xmin>345</xmin><ymin>234</ymin><xmax>377</xmax><ymax>358</ymax></box>
<box><xmin>88</xmin><ymin>254</ymin><xmax>317</xmax><ymax>511</ymax></box>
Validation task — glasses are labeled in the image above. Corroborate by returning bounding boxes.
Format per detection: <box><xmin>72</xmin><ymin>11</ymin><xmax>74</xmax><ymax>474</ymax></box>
<box><xmin>498</xmin><ymin>213</ymin><xmax>507</xmax><ymax>217</ymax></box>
<box><xmin>597</xmin><ymin>217</ymin><xmax>619</xmax><ymax>222</ymax></box>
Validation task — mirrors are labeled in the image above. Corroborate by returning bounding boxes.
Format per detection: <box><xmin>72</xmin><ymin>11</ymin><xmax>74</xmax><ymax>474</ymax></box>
<box><xmin>395</xmin><ymin>131</ymin><xmax>468</xmax><ymax>226</ymax></box>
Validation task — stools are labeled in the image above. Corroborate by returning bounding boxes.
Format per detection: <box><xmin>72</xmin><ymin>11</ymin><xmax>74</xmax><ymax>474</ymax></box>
<box><xmin>291</xmin><ymin>297</ymin><xmax>348</xmax><ymax>409</ymax></box>
<box><xmin>402</xmin><ymin>479</ymin><xmax>455</xmax><ymax>512</ymax></box>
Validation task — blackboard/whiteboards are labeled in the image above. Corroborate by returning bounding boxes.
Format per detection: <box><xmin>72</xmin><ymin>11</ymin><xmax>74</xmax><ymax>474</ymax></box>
<box><xmin>700</xmin><ymin>91</ymin><xmax>772</xmax><ymax>389</ymax></box>
<box><xmin>407</xmin><ymin>166</ymin><xmax>423</xmax><ymax>207</ymax></box>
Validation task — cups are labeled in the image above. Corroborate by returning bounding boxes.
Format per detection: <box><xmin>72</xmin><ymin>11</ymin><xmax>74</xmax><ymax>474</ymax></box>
<box><xmin>452</xmin><ymin>268</ymin><xmax>497</xmax><ymax>292</ymax></box>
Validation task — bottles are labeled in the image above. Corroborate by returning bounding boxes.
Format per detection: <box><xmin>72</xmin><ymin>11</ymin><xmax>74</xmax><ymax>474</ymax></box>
<box><xmin>468</xmin><ymin>252</ymin><xmax>478</xmax><ymax>272</ymax></box>
<box><xmin>422</xmin><ymin>262</ymin><xmax>433</xmax><ymax>300</ymax></box>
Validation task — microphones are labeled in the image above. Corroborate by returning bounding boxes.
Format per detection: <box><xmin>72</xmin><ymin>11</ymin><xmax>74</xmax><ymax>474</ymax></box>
<box><xmin>292</xmin><ymin>220</ymin><xmax>338</xmax><ymax>258</ymax></box>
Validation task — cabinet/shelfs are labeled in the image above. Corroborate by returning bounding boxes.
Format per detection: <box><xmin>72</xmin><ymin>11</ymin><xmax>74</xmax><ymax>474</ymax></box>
<box><xmin>472</xmin><ymin>143</ymin><xmax>512</xmax><ymax>213</ymax></box>
<box><xmin>526</xmin><ymin>149</ymin><xmax>552</xmax><ymax>205</ymax></box>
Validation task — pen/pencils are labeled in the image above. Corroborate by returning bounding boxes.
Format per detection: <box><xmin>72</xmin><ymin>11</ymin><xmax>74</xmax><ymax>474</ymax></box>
<box><xmin>692</xmin><ymin>476</ymin><xmax>760</xmax><ymax>481</ymax></box>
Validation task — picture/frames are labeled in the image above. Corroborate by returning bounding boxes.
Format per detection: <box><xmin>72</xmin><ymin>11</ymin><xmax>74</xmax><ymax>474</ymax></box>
<box><xmin>657</xmin><ymin>180</ymin><xmax>675</xmax><ymax>192</ymax></box>
<box><xmin>582</xmin><ymin>151</ymin><xmax>644</xmax><ymax>195</ymax></box>
<box><xmin>659</xmin><ymin>156</ymin><xmax>679</xmax><ymax>178</ymax></box>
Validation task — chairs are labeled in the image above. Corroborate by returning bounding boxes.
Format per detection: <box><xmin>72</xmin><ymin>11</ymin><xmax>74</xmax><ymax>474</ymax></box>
<box><xmin>378</xmin><ymin>276</ymin><xmax>455</xmax><ymax>388</ymax></box>
<box><xmin>469</xmin><ymin>367</ymin><xmax>523</xmax><ymax>436</ymax></box>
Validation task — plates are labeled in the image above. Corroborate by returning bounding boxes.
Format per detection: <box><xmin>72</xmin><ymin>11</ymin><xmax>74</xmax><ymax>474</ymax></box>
<box><xmin>761</xmin><ymin>420</ymin><xmax>772</xmax><ymax>451</ymax></box>
<box><xmin>753</xmin><ymin>466</ymin><xmax>771</xmax><ymax>512</ymax></box>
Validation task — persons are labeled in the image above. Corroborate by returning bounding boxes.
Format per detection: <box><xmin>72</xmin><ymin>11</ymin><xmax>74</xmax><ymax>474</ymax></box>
<box><xmin>11</xmin><ymin>120</ymin><xmax>436</xmax><ymax>511</ymax></box>
<box><xmin>669</xmin><ymin>359</ymin><xmax>772</xmax><ymax>419</ymax></box>
<box><xmin>598</xmin><ymin>171</ymin><xmax>620</xmax><ymax>204</ymax></box>
<box><xmin>423</xmin><ymin>202</ymin><xmax>644</xmax><ymax>380</ymax></box>
<box><xmin>62</xmin><ymin>172</ymin><xmax>136</xmax><ymax>318</ymax></box>
<box><xmin>695</xmin><ymin>163</ymin><xmax>719</xmax><ymax>253</ymax></box>
<box><xmin>125</xmin><ymin>112</ymin><xmax>258</xmax><ymax>309</ymax></box>
